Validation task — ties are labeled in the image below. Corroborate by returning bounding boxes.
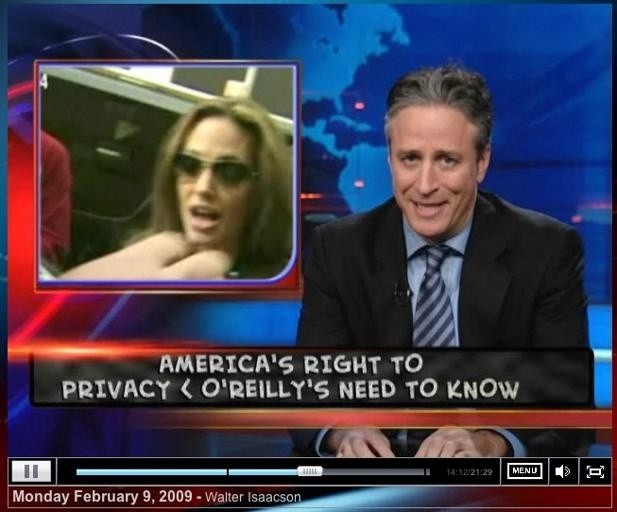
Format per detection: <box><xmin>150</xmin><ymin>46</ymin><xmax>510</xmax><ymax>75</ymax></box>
<box><xmin>411</xmin><ymin>242</ymin><xmax>458</xmax><ymax>352</ymax></box>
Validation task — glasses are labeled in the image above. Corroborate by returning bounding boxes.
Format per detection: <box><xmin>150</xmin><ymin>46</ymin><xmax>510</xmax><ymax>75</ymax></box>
<box><xmin>171</xmin><ymin>150</ymin><xmax>264</xmax><ymax>189</ymax></box>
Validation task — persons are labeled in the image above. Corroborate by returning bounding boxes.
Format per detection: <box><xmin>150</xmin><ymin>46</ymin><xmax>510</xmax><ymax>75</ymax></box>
<box><xmin>57</xmin><ymin>96</ymin><xmax>292</xmax><ymax>280</ymax></box>
<box><xmin>287</xmin><ymin>58</ymin><xmax>598</xmax><ymax>457</ymax></box>
